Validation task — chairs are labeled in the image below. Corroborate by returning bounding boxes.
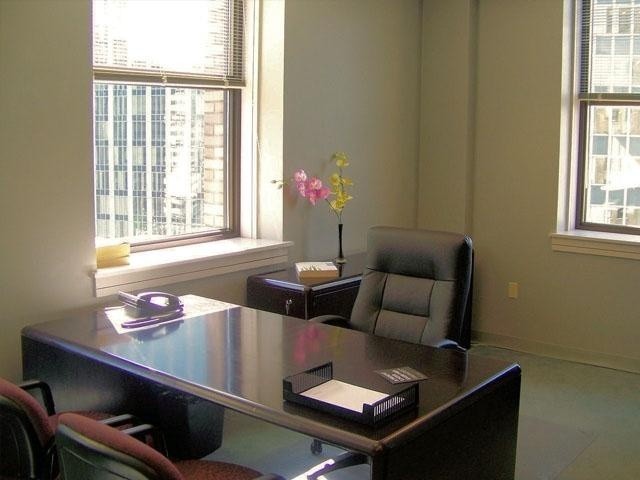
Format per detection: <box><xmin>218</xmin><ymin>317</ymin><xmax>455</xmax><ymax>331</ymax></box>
<box><xmin>1</xmin><ymin>375</ymin><xmax>271</xmax><ymax>480</ymax></box>
<box><xmin>308</xmin><ymin>225</ymin><xmax>474</xmax><ymax>353</ymax></box>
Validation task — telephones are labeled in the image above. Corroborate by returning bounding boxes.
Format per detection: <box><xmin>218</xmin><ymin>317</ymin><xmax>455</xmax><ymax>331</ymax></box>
<box><xmin>118</xmin><ymin>291</ymin><xmax>185</xmax><ymax>314</ymax></box>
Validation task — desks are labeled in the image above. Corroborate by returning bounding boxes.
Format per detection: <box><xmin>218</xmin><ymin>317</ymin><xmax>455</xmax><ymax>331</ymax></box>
<box><xmin>248</xmin><ymin>253</ymin><xmax>365</xmax><ymax>328</ymax></box>
<box><xmin>18</xmin><ymin>289</ymin><xmax>521</xmax><ymax>478</ymax></box>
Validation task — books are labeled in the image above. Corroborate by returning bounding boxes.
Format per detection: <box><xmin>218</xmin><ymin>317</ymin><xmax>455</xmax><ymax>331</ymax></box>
<box><xmin>295</xmin><ymin>262</ymin><xmax>338</xmax><ymax>279</ymax></box>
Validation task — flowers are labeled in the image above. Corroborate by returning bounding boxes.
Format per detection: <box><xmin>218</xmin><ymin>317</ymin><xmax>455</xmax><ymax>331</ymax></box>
<box><xmin>272</xmin><ymin>152</ymin><xmax>356</xmax><ymax>223</ymax></box>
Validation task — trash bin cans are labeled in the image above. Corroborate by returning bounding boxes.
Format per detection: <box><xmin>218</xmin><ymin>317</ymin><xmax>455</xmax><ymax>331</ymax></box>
<box><xmin>125</xmin><ymin>372</ymin><xmax>223</xmax><ymax>460</ymax></box>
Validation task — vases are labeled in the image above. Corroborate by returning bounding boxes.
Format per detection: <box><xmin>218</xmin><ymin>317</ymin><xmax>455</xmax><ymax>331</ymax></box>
<box><xmin>336</xmin><ymin>224</ymin><xmax>347</xmax><ymax>263</ymax></box>
